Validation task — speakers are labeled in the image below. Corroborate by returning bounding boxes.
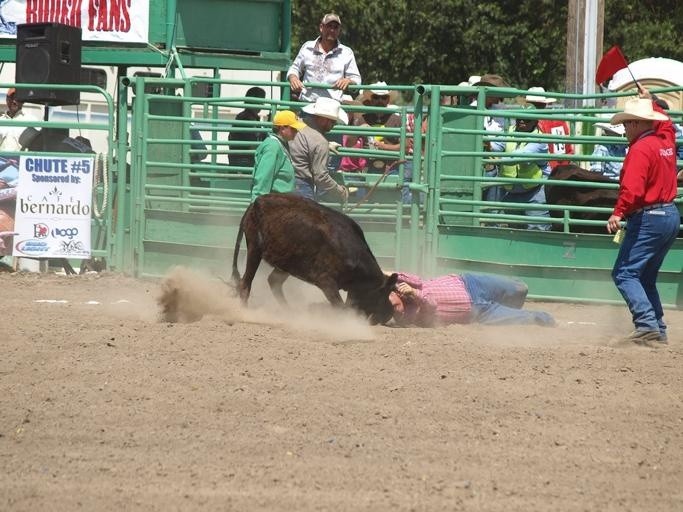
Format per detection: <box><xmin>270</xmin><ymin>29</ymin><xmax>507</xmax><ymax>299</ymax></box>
<box><xmin>15</xmin><ymin>22</ymin><xmax>81</xmax><ymax>106</ymax></box>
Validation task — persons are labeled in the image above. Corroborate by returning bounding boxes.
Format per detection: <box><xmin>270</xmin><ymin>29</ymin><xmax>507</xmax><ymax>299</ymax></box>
<box><xmin>338</xmin><ymin>80</ymin><xmax>428</xmax><ymax>208</ymax></box>
<box><xmin>284</xmin><ymin>12</ymin><xmax>362</xmax><ymax>178</ymax></box>
<box><xmin>458</xmin><ymin>75</ymin><xmax>574</xmax><ymax>232</ymax></box>
<box><xmin>188</xmin><ymin>122</ymin><xmax>208</xmax><ymax>188</ymax></box>
<box><xmin>227</xmin><ymin>88</ymin><xmax>349</xmax><ymax>207</ymax></box>
<box><xmin>380</xmin><ymin>267</ymin><xmax>557</xmax><ymax>328</ymax></box>
<box><xmin>0</xmin><ymin>88</ymin><xmax>130</xmax><ymax>257</ymax></box>
<box><xmin>590</xmin><ymin>82</ymin><xmax>683</xmax><ymax>345</ymax></box>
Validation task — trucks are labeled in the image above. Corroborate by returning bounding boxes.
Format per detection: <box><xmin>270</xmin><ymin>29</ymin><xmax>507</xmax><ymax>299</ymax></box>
<box><xmin>0</xmin><ymin>60</ymin><xmax>282</xmax><ymax>169</ymax></box>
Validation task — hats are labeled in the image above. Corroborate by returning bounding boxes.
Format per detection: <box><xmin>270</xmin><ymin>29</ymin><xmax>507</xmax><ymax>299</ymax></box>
<box><xmin>369</xmin><ymin>80</ymin><xmax>392</xmax><ymax>96</ymax></box>
<box><xmin>593</xmin><ymin>98</ymin><xmax>669</xmax><ymax>139</ymax></box>
<box><xmin>525</xmin><ymin>85</ymin><xmax>558</xmax><ymax>104</ymax></box>
<box><xmin>341</xmin><ymin>92</ymin><xmax>365</xmax><ymax>118</ymax></box>
<box><xmin>459</xmin><ymin>74</ymin><xmax>508</xmax><ymax>98</ymax></box>
<box><xmin>322</xmin><ymin>13</ymin><xmax>342</xmax><ymax>25</ymax></box>
<box><xmin>301</xmin><ymin>97</ymin><xmax>350</xmax><ymax>125</ymax></box>
<box><xmin>272</xmin><ymin>109</ymin><xmax>306</xmax><ymax>130</ymax></box>
<box><xmin>7</xmin><ymin>86</ymin><xmax>17</xmax><ymax>96</ymax></box>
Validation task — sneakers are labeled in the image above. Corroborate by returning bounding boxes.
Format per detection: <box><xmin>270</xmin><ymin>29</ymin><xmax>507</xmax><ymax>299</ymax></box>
<box><xmin>625</xmin><ymin>328</ymin><xmax>662</xmax><ymax>342</ymax></box>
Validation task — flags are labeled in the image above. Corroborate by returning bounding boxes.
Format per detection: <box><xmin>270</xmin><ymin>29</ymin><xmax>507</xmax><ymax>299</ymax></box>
<box><xmin>596</xmin><ymin>46</ymin><xmax>628</xmax><ymax>85</ymax></box>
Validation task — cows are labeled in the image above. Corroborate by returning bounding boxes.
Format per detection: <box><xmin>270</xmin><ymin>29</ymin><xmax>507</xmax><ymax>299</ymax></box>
<box><xmin>230</xmin><ymin>192</ymin><xmax>398</xmax><ymax>326</ymax></box>
<box><xmin>544</xmin><ymin>165</ymin><xmax>683</xmax><ymax>239</ymax></box>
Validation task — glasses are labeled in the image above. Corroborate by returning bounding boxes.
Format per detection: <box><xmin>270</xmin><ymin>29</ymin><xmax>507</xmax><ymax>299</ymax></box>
<box><xmin>370</xmin><ymin>93</ymin><xmax>389</xmax><ymax>101</ymax></box>
